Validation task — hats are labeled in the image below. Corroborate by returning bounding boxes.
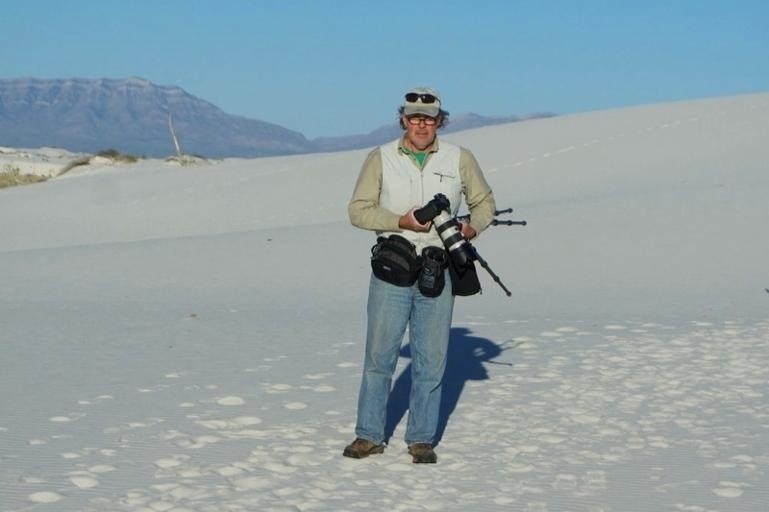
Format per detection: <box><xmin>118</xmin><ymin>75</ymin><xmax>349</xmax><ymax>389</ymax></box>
<box><xmin>403</xmin><ymin>88</ymin><xmax>441</xmax><ymax>118</ymax></box>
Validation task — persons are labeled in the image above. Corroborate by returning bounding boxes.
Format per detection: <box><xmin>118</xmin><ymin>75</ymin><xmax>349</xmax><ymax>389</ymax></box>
<box><xmin>340</xmin><ymin>85</ymin><xmax>499</xmax><ymax>464</ymax></box>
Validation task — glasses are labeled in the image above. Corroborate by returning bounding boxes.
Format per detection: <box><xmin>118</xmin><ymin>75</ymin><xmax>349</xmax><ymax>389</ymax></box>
<box><xmin>404</xmin><ymin>92</ymin><xmax>440</xmax><ymax>103</ymax></box>
<box><xmin>408</xmin><ymin>115</ymin><xmax>437</xmax><ymax>126</ymax></box>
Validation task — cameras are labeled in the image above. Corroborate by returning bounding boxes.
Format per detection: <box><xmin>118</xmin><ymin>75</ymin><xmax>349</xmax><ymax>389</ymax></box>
<box><xmin>413</xmin><ymin>193</ymin><xmax>474</xmax><ymax>276</ymax></box>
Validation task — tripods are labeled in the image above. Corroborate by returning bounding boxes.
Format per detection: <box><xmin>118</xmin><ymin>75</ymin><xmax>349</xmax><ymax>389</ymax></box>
<box><xmin>457</xmin><ymin>208</ymin><xmax>527</xmax><ymax>296</ymax></box>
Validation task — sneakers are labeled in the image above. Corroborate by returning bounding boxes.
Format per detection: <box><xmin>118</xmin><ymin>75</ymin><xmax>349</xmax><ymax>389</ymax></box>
<box><xmin>343</xmin><ymin>438</ymin><xmax>384</xmax><ymax>458</ymax></box>
<box><xmin>406</xmin><ymin>442</ymin><xmax>437</xmax><ymax>463</ymax></box>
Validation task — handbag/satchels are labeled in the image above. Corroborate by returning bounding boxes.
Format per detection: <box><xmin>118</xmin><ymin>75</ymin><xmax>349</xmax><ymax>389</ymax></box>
<box><xmin>370</xmin><ymin>235</ymin><xmax>420</xmax><ymax>287</ymax></box>
